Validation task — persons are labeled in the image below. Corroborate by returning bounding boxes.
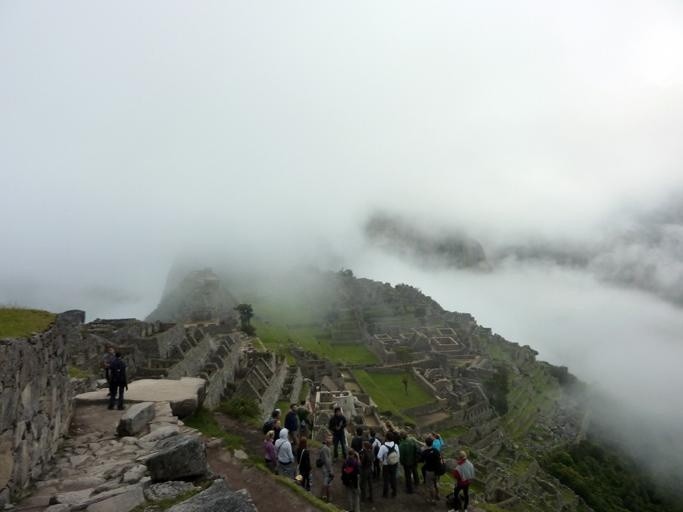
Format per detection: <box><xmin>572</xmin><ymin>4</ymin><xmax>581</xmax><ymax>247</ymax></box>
<box><xmin>103</xmin><ymin>347</ymin><xmax>117</xmax><ymax>396</ymax></box>
<box><xmin>262</xmin><ymin>400</ymin><xmax>476</xmax><ymax>512</ymax></box>
<box><xmin>107</xmin><ymin>351</ymin><xmax>128</xmax><ymax>410</ymax></box>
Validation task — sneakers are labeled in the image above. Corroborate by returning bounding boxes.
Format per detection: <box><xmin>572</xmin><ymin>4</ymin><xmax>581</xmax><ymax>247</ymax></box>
<box><xmin>447</xmin><ymin>509</ymin><xmax>469</xmax><ymax>512</ymax></box>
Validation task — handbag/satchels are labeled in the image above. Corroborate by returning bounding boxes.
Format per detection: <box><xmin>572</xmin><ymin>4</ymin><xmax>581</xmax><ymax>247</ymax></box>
<box><xmin>293</xmin><ymin>474</ymin><xmax>305</xmax><ymax>483</ymax></box>
<box><xmin>437</xmin><ymin>455</ymin><xmax>447</xmax><ymax>477</ymax></box>
<box><xmin>343</xmin><ymin>460</ymin><xmax>354</xmax><ymax>474</ymax></box>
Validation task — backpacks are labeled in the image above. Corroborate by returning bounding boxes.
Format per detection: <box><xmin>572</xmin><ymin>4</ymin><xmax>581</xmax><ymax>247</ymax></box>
<box><xmin>385</xmin><ymin>443</ymin><xmax>399</xmax><ymax>465</ymax></box>
